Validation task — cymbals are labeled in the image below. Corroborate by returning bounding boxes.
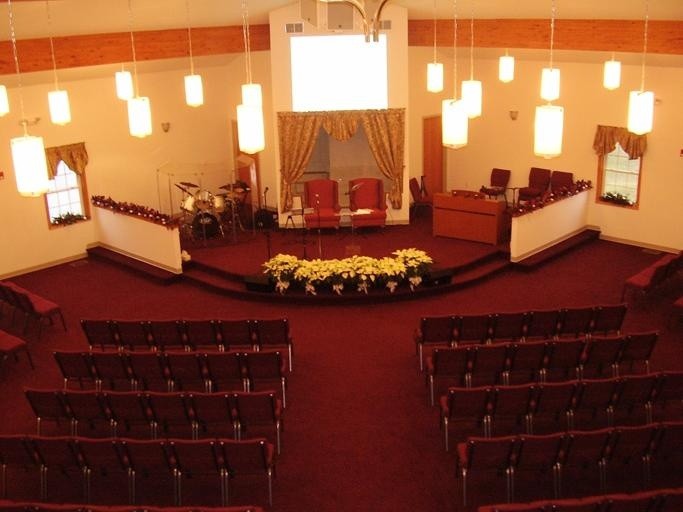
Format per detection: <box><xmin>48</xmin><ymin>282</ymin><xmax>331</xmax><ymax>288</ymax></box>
<box><xmin>175</xmin><ymin>184</ymin><xmax>193</xmax><ymax>196</ymax></box>
<box><xmin>180</xmin><ymin>182</ymin><xmax>199</xmax><ymax>187</ymax></box>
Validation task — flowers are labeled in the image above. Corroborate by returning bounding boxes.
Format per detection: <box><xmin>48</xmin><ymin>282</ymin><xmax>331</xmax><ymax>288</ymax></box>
<box><xmin>259</xmin><ymin>248</ymin><xmax>434</xmax><ymax>297</ymax></box>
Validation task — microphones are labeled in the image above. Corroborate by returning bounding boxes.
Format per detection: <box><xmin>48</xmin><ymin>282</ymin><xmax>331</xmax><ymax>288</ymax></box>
<box><xmin>263</xmin><ymin>186</ymin><xmax>269</xmax><ymax>195</ymax></box>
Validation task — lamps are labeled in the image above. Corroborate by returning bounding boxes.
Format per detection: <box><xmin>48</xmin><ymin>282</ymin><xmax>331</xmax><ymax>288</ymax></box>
<box><xmin>237</xmin><ymin>1</ymin><xmax>265</xmax><ymax>155</ymax></box>
<box><xmin>115</xmin><ymin>1</ymin><xmax>154</xmax><ymax>139</ymax></box>
<box><xmin>427</xmin><ymin>1</ymin><xmax>654</xmax><ymax>157</ymax></box>
<box><xmin>7</xmin><ymin>2</ymin><xmax>48</xmax><ymax>198</ymax></box>
<box><xmin>182</xmin><ymin>0</ymin><xmax>204</xmax><ymax>108</ymax></box>
<box><xmin>44</xmin><ymin>0</ymin><xmax>72</xmax><ymax>126</ymax></box>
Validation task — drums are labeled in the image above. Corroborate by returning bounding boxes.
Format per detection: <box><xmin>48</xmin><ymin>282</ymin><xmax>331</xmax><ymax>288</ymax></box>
<box><xmin>180</xmin><ymin>195</ymin><xmax>198</xmax><ymax>215</ymax></box>
<box><xmin>214</xmin><ymin>194</ymin><xmax>233</xmax><ymax>213</ymax></box>
<box><xmin>194</xmin><ymin>189</ymin><xmax>212</xmax><ymax>210</ymax></box>
<box><xmin>192</xmin><ymin>211</ymin><xmax>221</xmax><ymax>240</ymax></box>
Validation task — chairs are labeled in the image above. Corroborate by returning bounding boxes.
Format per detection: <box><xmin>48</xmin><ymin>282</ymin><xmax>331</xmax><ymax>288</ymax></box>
<box><xmin>348</xmin><ymin>177</ymin><xmax>388</xmax><ymax>235</ymax></box>
<box><xmin>0</xmin><ymin>278</ymin><xmax>66</xmax><ymax>370</ymax></box>
<box><xmin>619</xmin><ymin>250</ymin><xmax>683</xmax><ymax>331</ymax></box>
<box><xmin>414</xmin><ymin>305</ymin><xmax>683</xmax><ymax>512</ymax></box>
<box><xmin>411</xmin><ymin>168</ymin><xmax>574</xmax><ymax>220</ymax></box>
<box><xmin>1</xmin><ymin>316</ymin><xmax>292</xmax><ymax>512</ymax></box>
<box><xmin>298</xmin><ymin>179</ymin><xmax>342</xmax><ymax>234</ymax></box>
